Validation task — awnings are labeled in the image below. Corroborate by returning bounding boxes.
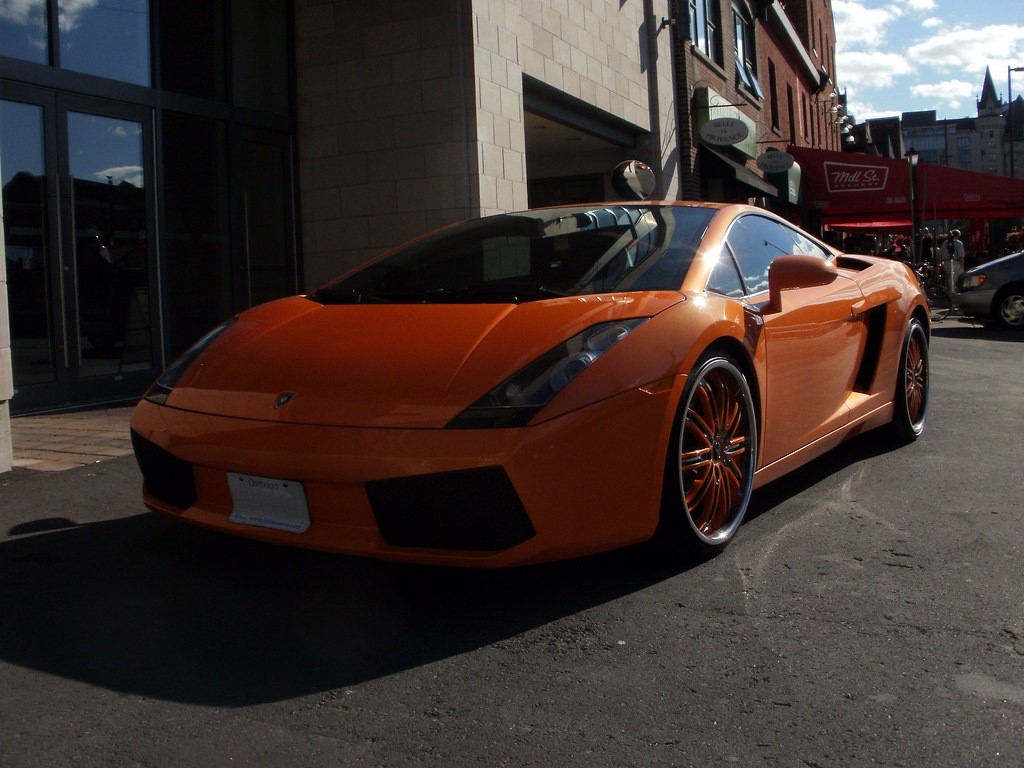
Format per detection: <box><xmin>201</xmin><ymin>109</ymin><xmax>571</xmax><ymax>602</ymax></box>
<box><xmin>822</xmin><ymin>216</ymin><xmax>912</xmax><ymax>231</ymax></box>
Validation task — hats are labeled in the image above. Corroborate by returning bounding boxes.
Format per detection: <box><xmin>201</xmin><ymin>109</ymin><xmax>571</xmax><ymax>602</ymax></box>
<box><xmin>898</xmin><ymin>235</ymin><xmax>903</xmax><ymax>240</ymax></box>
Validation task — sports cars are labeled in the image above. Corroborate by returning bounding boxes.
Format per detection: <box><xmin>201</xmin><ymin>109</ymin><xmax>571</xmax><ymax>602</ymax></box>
<box><xmin>128</xmin><ymin>200</ymin><xmax>934</xmax><ymax>568</ymax></box>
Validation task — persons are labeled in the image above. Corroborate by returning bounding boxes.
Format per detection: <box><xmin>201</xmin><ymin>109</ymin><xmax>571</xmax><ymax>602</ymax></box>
<box><xmin>843</xmin><ymin>227</ymin><xmax>948</xmax><ymax>274</ymax></box>
<box><xmin>940</xmin><ymin>229</ymin><xmax>965</xmax><ymax>308</ymax></box>
<box><xmin>1006</xmin><ymin>226</ymin><xmax>1024</xmax><ymax>250</ymax></box>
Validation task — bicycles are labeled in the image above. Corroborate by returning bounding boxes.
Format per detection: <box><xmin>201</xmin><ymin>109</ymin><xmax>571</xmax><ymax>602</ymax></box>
<box><xmin>915</xmin><ymin>259</ymin><xmax>952</xmax><ymax>323</ymax></box>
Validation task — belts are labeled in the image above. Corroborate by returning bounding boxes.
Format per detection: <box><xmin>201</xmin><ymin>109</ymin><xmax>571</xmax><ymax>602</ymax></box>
<box><xmin>946</xmin><ymin>258</ymin><xmax>960</xmax><ymax>261</ymax></box>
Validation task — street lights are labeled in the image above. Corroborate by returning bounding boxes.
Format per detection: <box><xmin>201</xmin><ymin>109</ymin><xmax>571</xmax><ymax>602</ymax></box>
<box><xmin>1008</xmin><ymin>65</ymin><xmax>1024</xmax><ymax>179</ymax></box>
<box><xmin>904</xmin><ymin>147</ymin><xmax>920</xmax><ymax>268</ymax></box>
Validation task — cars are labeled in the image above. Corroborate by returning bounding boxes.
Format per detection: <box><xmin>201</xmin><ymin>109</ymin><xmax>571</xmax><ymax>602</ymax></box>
<box><xmin>948</xmin><ymin>249</ymin><xmax>1024</xmax><ymax>340</ymax></box>
